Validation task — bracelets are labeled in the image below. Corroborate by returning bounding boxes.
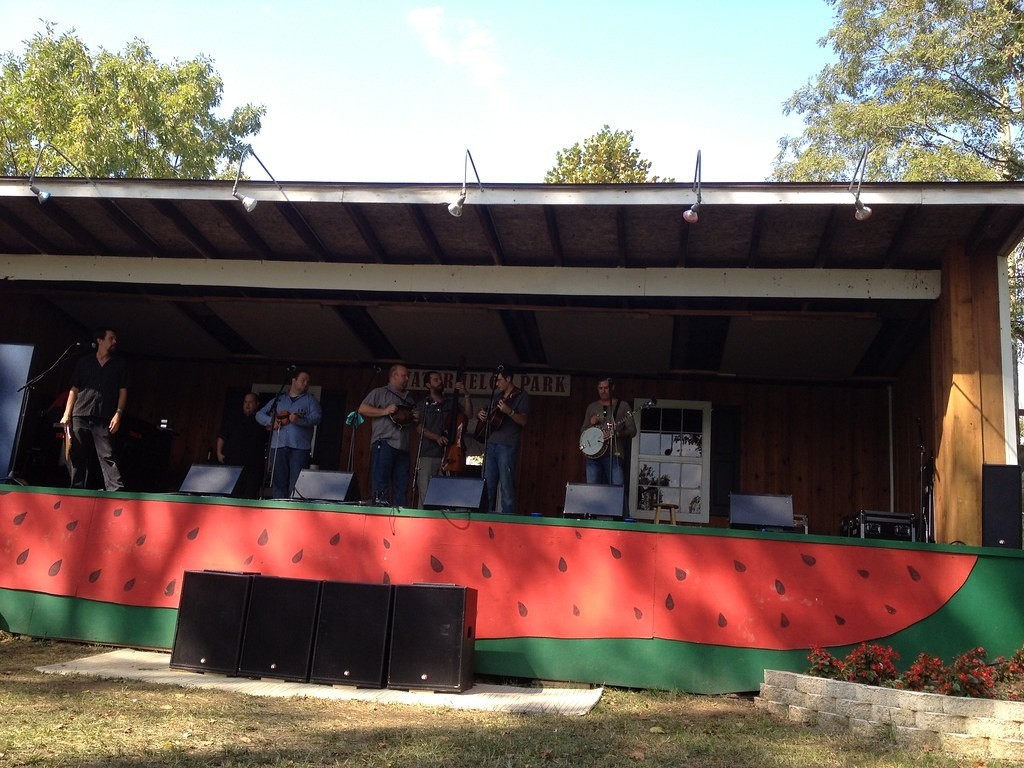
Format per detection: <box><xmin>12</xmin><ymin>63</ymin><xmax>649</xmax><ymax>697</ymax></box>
<box><xmin>116</xmin><ymin>408</ymin><xmax>122</xmax><ymax>413</ymax></box>
<box><xmin>508</xmin><ymin>409</ymin><xmax>514</xmax><ymax>416</ymax></box>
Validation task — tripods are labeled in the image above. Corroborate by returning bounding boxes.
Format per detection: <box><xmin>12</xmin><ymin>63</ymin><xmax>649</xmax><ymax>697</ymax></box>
<box><xmin>0</xmin><ymin>344</ymin><xmax>89</xmax><ymax>487</ymax></box>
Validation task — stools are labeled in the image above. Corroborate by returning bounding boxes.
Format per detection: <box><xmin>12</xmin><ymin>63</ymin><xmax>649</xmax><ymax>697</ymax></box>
<box><xmin>655</xmin><ymin>502</ymin><xmax>677</xmax><ymax>526</ymax></box>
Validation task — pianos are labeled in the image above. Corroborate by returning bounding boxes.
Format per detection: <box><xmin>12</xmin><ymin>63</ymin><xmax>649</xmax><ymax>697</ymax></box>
<box><xmin>52</xmin><ymin>406</ymin><xmax>180</xmax><ymax>488</ymax></box>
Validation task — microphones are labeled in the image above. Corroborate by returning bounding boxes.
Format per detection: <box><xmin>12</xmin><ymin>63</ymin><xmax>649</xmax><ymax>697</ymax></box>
<box><xmin>373</xmin><ymin>365</ymin><xmax>382</xmax><ymax>373</ymax></box>
<box><xmin>425</xmin><ymin>396</ymin><xmax>431</xmax><ymax>406</ymax></box>
<box><xmin>496</xmin><ymin>365</ymin><xmax>504</xmax><ymax>371</ymax></box>
<box><xmin>74</xmin><ymin>342</ymin><xmax>96</xmax><ymax>348</ymax></box>
<box><xmin>286</xmin><ymin>364</ymin><xmax>296</xmax><ymax>371</ymax></box>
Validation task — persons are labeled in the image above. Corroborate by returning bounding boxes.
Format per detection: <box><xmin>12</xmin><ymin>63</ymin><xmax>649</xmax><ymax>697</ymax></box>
<box><xmin>58</xmin><ymin>327</ymin><xmax>129</xmax><ymax>493</ymax></box>
<box><xmin>413</xmin><ymin>371</ymin><xmax>473</xmax><ymax>511</ymax></box>
<box><xmin>254</xmin><ymin>370</ymin><xmax>321</xmax><ymax>499</ymax></box>
<box><xmin>215</xmin><ymin>392</ymin><xmax>268</xmax><ymax>499</ymax></box>
<box><xmin>475</xmin><ymin>368</ymin><xmax>530</xmax><ymax>516</ymax></box>
<box><xmin>580</xmin><ymin>378</ymin><xmax>637</xmax><ymax>520</ymax></box>
<box><xmin>357</xmin><ymin>364</ymin><xmax>421</xmax><ymax>508</ymax></box>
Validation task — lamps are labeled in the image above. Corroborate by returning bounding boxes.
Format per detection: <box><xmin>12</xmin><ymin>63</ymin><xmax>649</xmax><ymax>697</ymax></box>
<box><xmin>28</xmin><ymin>144</ymin><xmax>96</xmax><ymax>205</ymax></box>
<box><xmin>847</xmin><ymin>143</ymin><xmax>872</xmax><ymax>220</ymax></box>
<box><xmin>448</xmin><ymin>149</ymin><xmax>483</xmax><ymax>217</ymax></box>
<box><xmin>232</xmin><ymin>148</ymin><xmax>281</xmax><ymax>212</ymax></box>
<box><xmin>683</xmin><ymin>150</ymin><xmax>702</xmax><ymax>222</ymax></box>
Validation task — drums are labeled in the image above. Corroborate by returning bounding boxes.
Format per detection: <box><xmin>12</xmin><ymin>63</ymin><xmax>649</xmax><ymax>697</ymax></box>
<box><xmin>388</xmin><ymin>404</ymin><xmax>415</xmax><ymax>426</ymax></box>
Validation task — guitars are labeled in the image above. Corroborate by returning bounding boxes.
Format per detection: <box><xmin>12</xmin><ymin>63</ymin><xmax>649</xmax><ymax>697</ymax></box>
<box><xmin>473</xmin><ymin>389</ymin><xmax>523</xmax><ymax>444</ymax></box>
<box><xmin>578</xmin><ymin>395</ymin><xmax>658</xmax><ymax>460</ymax></box>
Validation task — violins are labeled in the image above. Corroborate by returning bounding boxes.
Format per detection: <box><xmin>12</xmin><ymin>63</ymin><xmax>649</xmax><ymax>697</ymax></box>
<box><xmin>266</xmin><ymin>408</ymin><xmax>307</xmax><ymax>432</ymax></box>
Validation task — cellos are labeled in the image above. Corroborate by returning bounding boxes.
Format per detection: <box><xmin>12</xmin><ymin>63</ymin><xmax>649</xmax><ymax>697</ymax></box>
<box><xmin>440</xmin><ymin>361</ymin><xmax>469</xmax><ymax>463</ymax></box>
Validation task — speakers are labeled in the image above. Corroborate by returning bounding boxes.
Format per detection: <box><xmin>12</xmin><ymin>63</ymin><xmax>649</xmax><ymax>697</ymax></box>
<box><xmin>982</xmin><ymin>465</ymin><xmax>1023</xmax><ymax>549</ymax></box>
<box><xmin>729</xmin><ymin>491</ymin><xmax>793</xmax><ymax>529</ymax></box>
<box><xmin>423</xmin><ymin>475</ymin><xmax>489</xmax><ymax>513</ymax></box>
<box><xmin>179</xmin><ymin>464</ymin><xmax>254</xmax><ymax>499</ymax></box>
<box><xmin>563</xmin><ymin>482</ymin><xmax>625</xmax><ymax>520</ymax></box>
<box><xmin>169</xmin><ymin>569</ymin><xmax>478</xmax><ymax>692</ymax></box>
<box><xmin>0</xmin><ymin>344</ymin><xmax>58</xmax><ymax>485</ymax></box>
<box><xmin>290</xmin><ymin>468</ymin><xmax>362</xmax><ymax>502</ymax></box>
